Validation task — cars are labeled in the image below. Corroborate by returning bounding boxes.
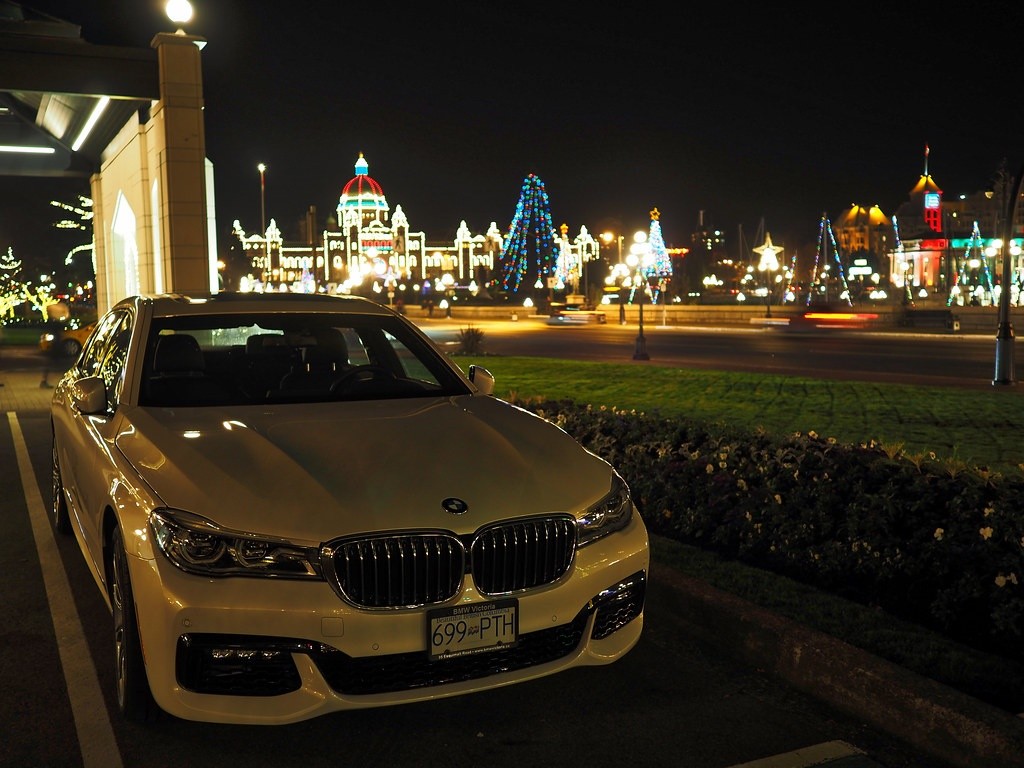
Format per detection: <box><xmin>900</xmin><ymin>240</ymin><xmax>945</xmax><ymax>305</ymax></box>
<box><xmin>49</xmin><ymin>293</ymin><xmax>648</xmax><ymax>727</ymax></box>
<box><xmin>39</xmin><ymin>322</ymin><xmax>96</xmax><ymax>359</ymax></box>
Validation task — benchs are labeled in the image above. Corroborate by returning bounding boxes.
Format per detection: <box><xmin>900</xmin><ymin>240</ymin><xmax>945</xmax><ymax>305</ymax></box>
<box><xmin>899</xmin><ymin>308</ymin><xmax>955</xmax><ymax>330</ymax></box>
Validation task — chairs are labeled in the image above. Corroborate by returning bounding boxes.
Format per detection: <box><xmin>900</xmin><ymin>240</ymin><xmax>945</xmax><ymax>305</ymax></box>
<box><xmin>146</xmin><ymin>328</ymin><xmax>415</xmax><ymax>411</ymax></box>
